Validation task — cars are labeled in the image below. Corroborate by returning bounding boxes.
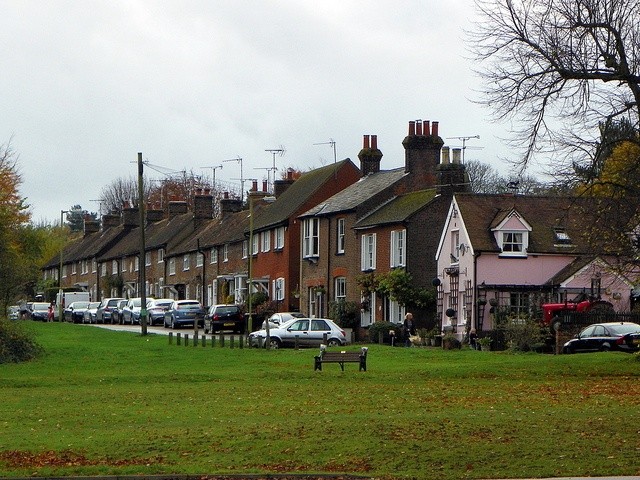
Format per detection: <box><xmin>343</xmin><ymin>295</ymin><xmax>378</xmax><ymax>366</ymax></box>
<box><xmin>110</xmin><ymin>300</ymin><xmax>128</xmax><ymax>324</ymax></box>
<box><xmin>562</xmin><ymin>322</ymin><xmax>640</xmax><ymax>354</ymax></box>
<box><xmin>248</xmin><ymin>317</ymin><xmax>346</xmax><ymax>349</ymax></box>
<box><xmin>262</xmin><ymin>313</ymin><xmax>307</xmax><ymax>329</ymax></box>
<box><xmin>82</xmin><ymin>302</ymin><xmax>100</xmax><ymax>324</ymax></box>
<box><xmin>121</xmin><ymin>298</ymin><xmax>153</xmax><ymax>325</ymax></box>
<box><xmin>64</xmin><ymin>301</ymin><xmax>90</xmax><ymax>323</ymax></box>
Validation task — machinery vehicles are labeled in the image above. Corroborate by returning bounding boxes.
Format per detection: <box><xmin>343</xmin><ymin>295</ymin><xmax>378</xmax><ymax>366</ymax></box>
<box><xmin>541</xmin><ymin>287</ymin><xmax>618</xmax><ymax>334</ymax></box>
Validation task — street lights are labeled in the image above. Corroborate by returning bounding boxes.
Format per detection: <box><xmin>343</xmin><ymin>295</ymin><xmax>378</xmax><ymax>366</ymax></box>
<box><xmin>245</xmin><ymin>190</ymin><xmax>271</xmax><ymax>332</ymax></box>
<box><xmin>58</xmin><ymin>209</ymin><xmax>72</xmax><ymax>321</ymax></box>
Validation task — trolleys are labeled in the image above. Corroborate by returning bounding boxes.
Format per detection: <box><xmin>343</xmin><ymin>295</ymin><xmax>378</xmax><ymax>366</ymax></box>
<box><xmin>408</xmin><ymin>328</ymin><xmax>423</xmax><ymax>348</ymax></box>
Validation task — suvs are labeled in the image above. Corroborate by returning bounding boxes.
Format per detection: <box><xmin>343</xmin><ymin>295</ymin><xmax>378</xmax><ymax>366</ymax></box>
<box><xmin>29</xmin><ymin>302</ymin><xmax>51</xmax><ymax>322</ymax></box>
<box><xmin>163</xmin><ymin>299</ymin><xmax>206</xmax><ymax>329</ymax></box>
<box><xmin>204</xmin><ymin>304</ymin><xmax>246</xmax><ymax>334</ymax></box>
<box><xmin>96</xmin><ymin>298</ymin><xmax>126</xmax><ymax>323</ymax></box>
<box><xmin>139</xmin><ymin>299</ymin><xmax>175</xmax><ymax>325</ymax></box>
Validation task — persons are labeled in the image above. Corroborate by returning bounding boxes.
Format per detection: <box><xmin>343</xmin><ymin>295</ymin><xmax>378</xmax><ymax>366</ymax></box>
<box><xmin>16</xmin><ymin>296</ymin><xmax>28</xmax><ymax>320</ymax></box>
<box><xmin>404</xmin><ymin>313</ymin><xmax>416</xmax><ymax>347</ymax></box>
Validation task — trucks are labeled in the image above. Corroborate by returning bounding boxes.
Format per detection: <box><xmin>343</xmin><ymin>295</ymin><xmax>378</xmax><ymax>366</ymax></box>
<box><xmin>48</xmin><ymin>292</ymin><xmax>89</xmax><ymax>322</ymax></box>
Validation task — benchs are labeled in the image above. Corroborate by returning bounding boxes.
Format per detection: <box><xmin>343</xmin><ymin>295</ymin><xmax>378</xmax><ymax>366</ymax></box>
<box><xmin>314</xmin><ymin>347</ymin><xmax>368</xmax><ymax>373</ymax></box>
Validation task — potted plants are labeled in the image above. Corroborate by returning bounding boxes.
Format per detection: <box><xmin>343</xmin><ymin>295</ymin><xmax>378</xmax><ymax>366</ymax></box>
<box><xmin>425</xmin><ymin>332</ymin><xmax>430</xmax><ymax>346</ymax></box>
<box><xmin>315</xmin><ymin>287</ymin><xmax>326</xmax><ymax>297</ymax></box>
<box><xmin>475</xmin><ymin>339</ymin><xmax>482</xmax><ymax>350</ymax></box>
<box><xmin>419</xmin><ymin>331</ymin><xmax>426</xmax><ymax>345</ymax></box>
<box><xmin>430</xmin><ymin>332</ymin><xmax>436</xmax><ymax>346</ymax></box>
<box><xmin>477</xmin><ymin>337</ymin><xmax>493</xmax><ymax>351</ymax></box>
<box><xmin>329</xmin><ymin>299</ymin><xmax>357</xmax><ymax>343</ymax></box>
<box><xmin>292</xmin><ymin>291</ymin><xmax>301</xmax><ymax>298</ymax></box>
